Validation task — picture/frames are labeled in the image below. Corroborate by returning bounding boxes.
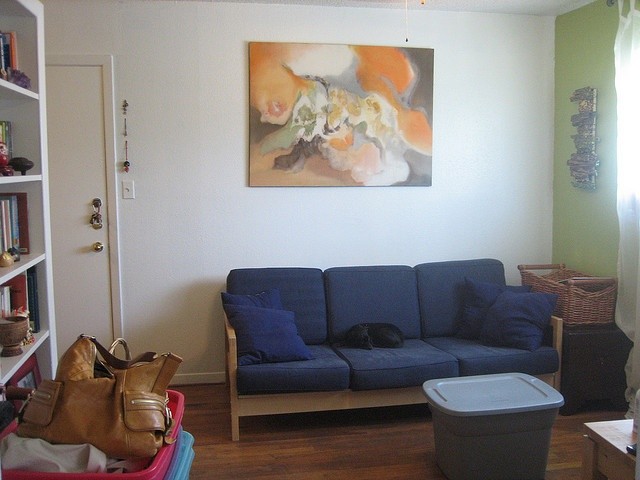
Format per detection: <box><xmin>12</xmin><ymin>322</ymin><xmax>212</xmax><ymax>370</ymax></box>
<box><xmin>249</xmin><ymin>42</ymin><xmax>434</xmax><ymax>186</ymax></box>
<box><xmin>5</xmin><ymin>353</ymin><xmax>43</xmax><ymax>415</ymax></box>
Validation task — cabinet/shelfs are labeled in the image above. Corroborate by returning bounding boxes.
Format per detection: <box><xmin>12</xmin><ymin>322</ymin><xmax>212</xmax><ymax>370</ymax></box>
<box><xmin>1</xmin><ymin>0</ymin><xmax>61</xmax><ymax>381</ymax></box>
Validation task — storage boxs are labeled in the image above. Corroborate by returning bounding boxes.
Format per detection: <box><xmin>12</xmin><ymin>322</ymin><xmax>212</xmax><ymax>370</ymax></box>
<box><xmin>167</xmin><ymin>424</ymin><xmax>194</xmax><ymax>479</ymax></box>
<box><xmin>1</xmin><ymin>390</ymin><xmax>183</xmax><ymax>479</ymax></box>
<box><xmin>422</xmin><ymin>372</ymin><xmax>565</xmax><ymax>480</ymax></box>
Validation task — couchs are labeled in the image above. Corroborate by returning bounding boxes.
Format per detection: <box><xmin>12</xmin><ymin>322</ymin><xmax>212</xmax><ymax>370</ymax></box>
<box><xmin>223</xmin><ymin>259</ymin><xmax>563</xmax><ymax>442</ymax></box>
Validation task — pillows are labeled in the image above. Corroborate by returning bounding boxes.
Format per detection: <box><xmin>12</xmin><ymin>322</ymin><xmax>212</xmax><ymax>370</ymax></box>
<box><xmin>220</xmin><ymin>287</ymin><xmax>282</xmax><ymax>309</ymax></box>
<box><xmin>224</xmin><ymin>305</ymin><xmax>317</xmax><ymax>366</ymax></box>
<box><xmin>480</xmin><ymin>289</ymin><xmax>558</xmax><ymax>352</ymax></box>
<box><xmin>455</xmin><ymin>276</ymin><xmax>533</xmax><ymax>340</ymax></box>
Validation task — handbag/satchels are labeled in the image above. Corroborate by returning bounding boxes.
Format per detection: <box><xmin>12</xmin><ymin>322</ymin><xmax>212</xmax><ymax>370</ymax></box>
<box><xmin>15</xmin><ymin>332</ymin><xmax>182</xmax><ymax>463</ymax></box>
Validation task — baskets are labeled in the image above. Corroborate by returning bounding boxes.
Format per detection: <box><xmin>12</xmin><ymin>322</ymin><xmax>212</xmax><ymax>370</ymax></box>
<box><xmin>517</xmin><ymin>263</ymin><xmax>618</xmax><ymax>328</ymax></box>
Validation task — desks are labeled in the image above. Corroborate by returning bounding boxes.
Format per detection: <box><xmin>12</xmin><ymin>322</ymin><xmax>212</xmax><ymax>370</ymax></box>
<box><xmin>559</xmin><ymin>322</ymin><xmax>625</xmax><ymax>416</ymax></box>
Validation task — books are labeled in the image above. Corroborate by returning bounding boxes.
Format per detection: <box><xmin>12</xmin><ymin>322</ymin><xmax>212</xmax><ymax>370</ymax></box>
<box><xmin>0</xmin><ymin>120</ymin><xmax>13</xmax><ymax>175</ymax></box>
<box><xmin>0</xmin><ymin>28</ymin><xmax>19</xmax><ymax>81</ymax></box>
<box><xmin>0</xmin><ymin>191</ymin><xmax>30</xmax><ymax>256</ymax></box>
<box><xmin>1</xmin><ymin>266</ymin><xmax>41</xmax><ymax>352</ymax></box>
